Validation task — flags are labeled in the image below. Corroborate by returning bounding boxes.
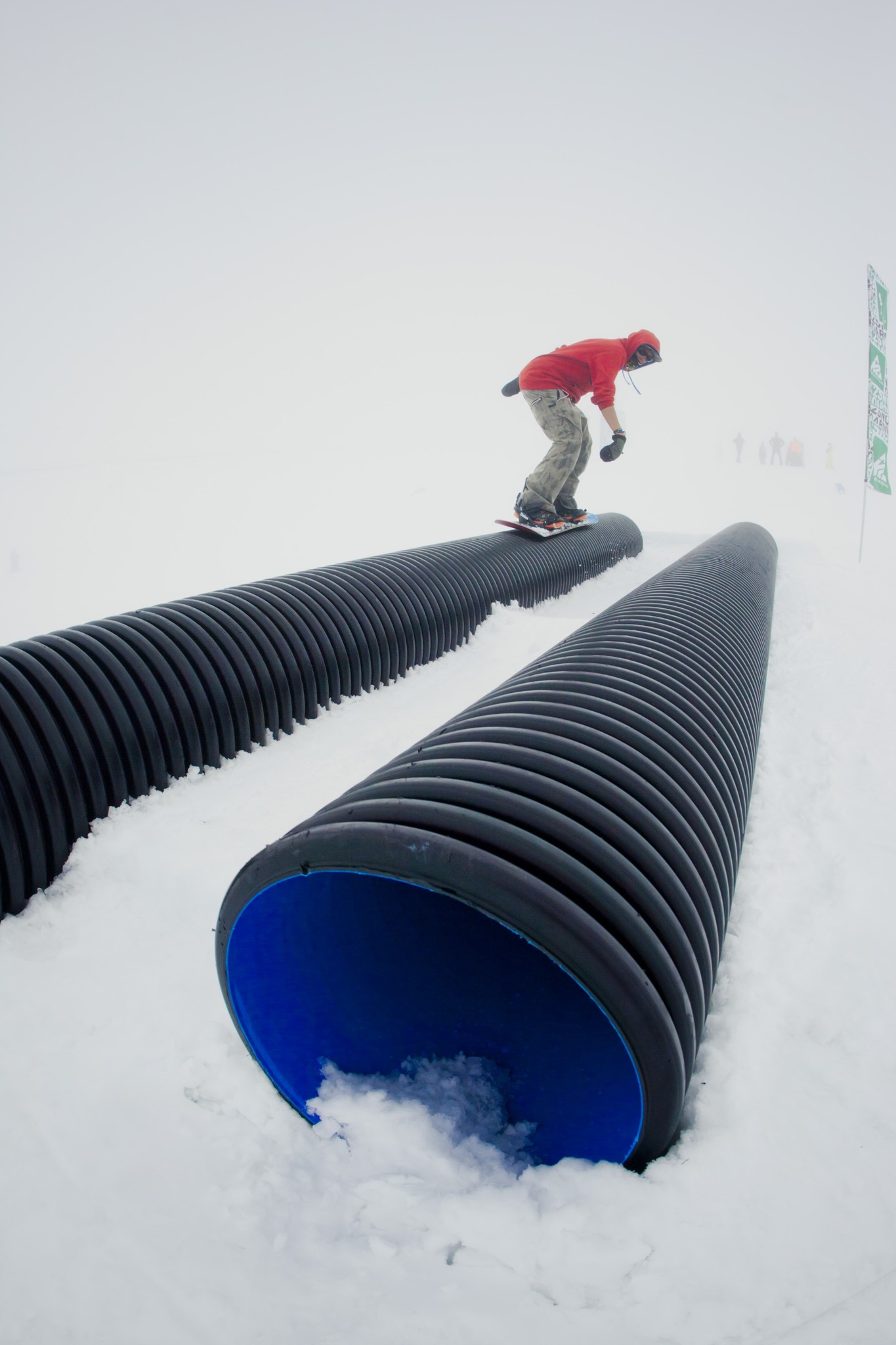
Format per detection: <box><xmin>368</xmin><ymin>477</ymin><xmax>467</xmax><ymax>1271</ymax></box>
<box><xmin>865</xmin><ymin>260</ymin><xmax>892</xmax><ymax>496</ymax></box>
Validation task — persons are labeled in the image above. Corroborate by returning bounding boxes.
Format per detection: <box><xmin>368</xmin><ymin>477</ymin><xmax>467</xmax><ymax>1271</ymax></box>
<box><xmin>501</xmin><ymin>329</ymin><xmax>662</xmax><ymax>528</ymax></box>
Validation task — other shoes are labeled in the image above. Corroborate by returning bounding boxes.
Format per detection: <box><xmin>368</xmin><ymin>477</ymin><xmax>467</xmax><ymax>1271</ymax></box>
<box><xmin>513</xmin><ymin>501</ymin><xmax>565</xmax><ymax>528</ymax></box>
<box><xmin>554</xmin><ymin>508</ymin><xmax>588</xmax><ymax>521</ymax></box>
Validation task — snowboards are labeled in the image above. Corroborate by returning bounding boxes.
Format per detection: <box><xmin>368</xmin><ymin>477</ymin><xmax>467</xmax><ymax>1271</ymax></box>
<box><xmin>495</xmin><ymin>507</ymin><xmax>601</xmax><ymax>539</ymax></box>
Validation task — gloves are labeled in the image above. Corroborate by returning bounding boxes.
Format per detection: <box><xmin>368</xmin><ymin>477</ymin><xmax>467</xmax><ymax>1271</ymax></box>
<box><xmin>600</xmin><ymin>434</ymin><xmax>627</xmax><ymax>462</ymax></box>
<box><xmin>501</xmin><ymin>376</ymin><xmax>521</xmax><ymax>397</ymax></box>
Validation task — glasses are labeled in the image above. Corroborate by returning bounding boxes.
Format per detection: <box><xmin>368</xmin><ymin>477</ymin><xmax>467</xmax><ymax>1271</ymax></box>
<box><xmin>637</xmin><ymin>346</ymin><xmax>653</xmax><ymax>363</ymax></box>
